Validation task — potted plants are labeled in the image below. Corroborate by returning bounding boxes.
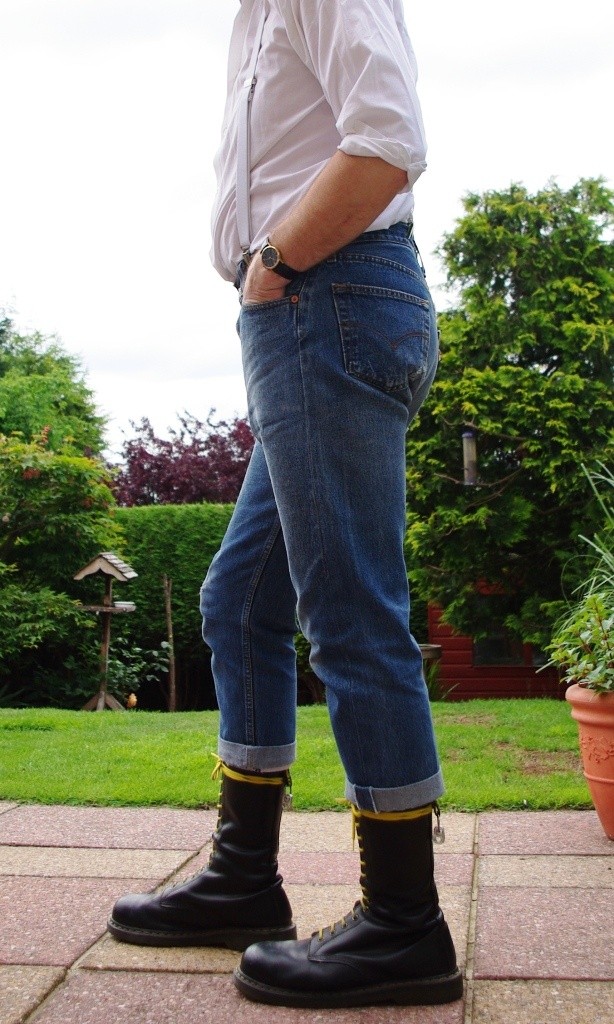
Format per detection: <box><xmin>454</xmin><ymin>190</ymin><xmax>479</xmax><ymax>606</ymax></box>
<box><xmin>543</xmin><ymin>577</ymin><xmax>614</xmax><ymax>842</ymax></box>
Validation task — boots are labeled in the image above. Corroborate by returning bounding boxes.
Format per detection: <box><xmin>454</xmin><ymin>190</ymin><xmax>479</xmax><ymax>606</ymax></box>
<box><xmin>106</xmin><ymin>754</ymin><xmax>299</xmax><ymax>950</ymax></box>
<box><xmin>232</xmin><ymin>795</ymin><xmax>464</xmax><ymax>1009</ymax></box>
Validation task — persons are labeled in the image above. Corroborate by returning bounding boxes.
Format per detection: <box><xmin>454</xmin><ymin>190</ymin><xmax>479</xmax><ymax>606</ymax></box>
<box><xmin>108</xmin><ymin>1</ymin><xmax>464</xmax><ymax>1008</ymax></box>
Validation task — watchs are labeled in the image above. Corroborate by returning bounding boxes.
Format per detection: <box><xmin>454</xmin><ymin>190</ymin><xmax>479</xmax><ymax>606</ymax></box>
<box><xmin>259</xmin><ymin>235</ymin><xmax>302</xmax><ymax>280</ymax></box>
